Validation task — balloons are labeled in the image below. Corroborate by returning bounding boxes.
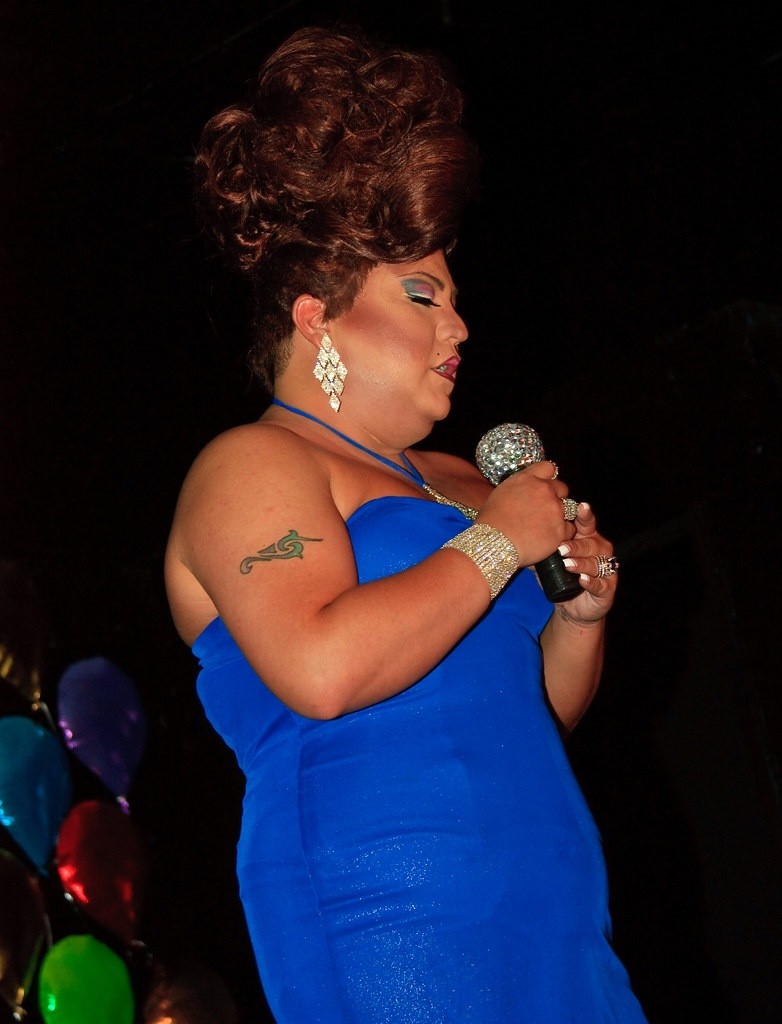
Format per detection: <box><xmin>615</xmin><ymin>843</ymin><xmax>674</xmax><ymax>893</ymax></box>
<box><xmin>38</xmin><ymin>935</ymin><xmax>134</xmax><ymax>1024</ymax></box>
<box><xmin>143</xmin><ymin>963</ymin><xmax>239</xmax><ymax>1024</ymax></box>
<box><xmin>0</xmin><ymin>715</ymin><xmax>71</xmax><ymax>876</ymax></box>
<box><xmin>0</xmin><ymin>846</ymin><xmax>45</xmax><ymax>1023</ymax></box>
<box><xmin>56</xmin><ymin>799</ymin><xmax>147</xmax><ymax>947</ymax></box>
<box><xmin>56</xmin><ymin>656</ymin><xmax>147</xmax><ymax>796</ymax></box>
<box><xmin>0</xmin><ymin>558</ymin><xmax>47</xmax><ymax>702</ymax></box>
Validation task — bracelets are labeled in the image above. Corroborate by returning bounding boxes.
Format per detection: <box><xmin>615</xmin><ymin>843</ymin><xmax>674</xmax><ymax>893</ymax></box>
<box><xmin>443</xmin><ymin>523</ymin><xmax>520</xmax><ymax>600</ymax></box>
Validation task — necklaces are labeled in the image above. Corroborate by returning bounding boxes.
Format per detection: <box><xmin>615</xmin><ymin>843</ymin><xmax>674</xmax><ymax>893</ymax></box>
<box><xmin>271</xmin><ymin>398</ymin><xmax>479</xmax><ymax>520</ymax></box>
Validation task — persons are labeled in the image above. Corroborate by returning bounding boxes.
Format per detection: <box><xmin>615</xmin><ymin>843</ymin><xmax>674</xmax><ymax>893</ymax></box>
<box><xmin>161</xmin><ymin>24</ymin><xmax>647</xmax><ymax>1024</ymax></box>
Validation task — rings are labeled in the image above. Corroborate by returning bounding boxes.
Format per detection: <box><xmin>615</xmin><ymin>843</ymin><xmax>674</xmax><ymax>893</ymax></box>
<box><xmin>550</xmin><ymin>460</ymin><xmax>559</xmax><ymax>480</ymax></box>
<box><xmin>597</xmin><ymin>555</ymin><xmax>618</xmax><ymax>577</ymax></box>
<box><xmin>563</xmin><ymin>497</ymin><xmax>578</xmax><ymax>520</ymax></box>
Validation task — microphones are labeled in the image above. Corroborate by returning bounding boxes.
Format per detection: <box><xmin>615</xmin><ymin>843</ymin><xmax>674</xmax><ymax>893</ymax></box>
<box><xmin>476</xmin><ymin>422</ymin><xmax>585</xmax><ymax>602</ymax></box>
